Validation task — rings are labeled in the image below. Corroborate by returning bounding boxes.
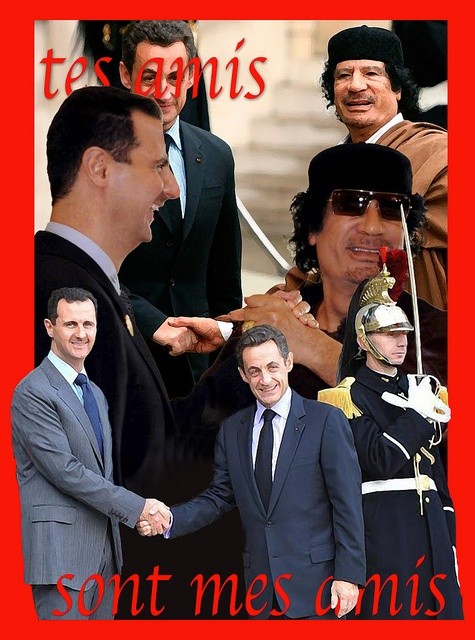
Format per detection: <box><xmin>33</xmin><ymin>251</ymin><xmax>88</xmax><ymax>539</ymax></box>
<box><xmin>242</xmin><ymin>321</ymin><xmax>255</xmax><ymax>332</ymax></box>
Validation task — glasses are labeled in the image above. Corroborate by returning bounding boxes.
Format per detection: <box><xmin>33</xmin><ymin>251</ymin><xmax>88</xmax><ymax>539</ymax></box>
<box><xmin>331</xmin><ymin>188</ymin><xmax>410</xmax><ymax>220</ymax></box>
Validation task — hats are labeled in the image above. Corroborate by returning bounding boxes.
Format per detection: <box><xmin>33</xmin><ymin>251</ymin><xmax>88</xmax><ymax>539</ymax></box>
<box><xmin>328</xmin><ymin>26</ymin><xmax>403</xmax><ymax>64</ymax></box>
<box><xmin>307</xmin><ymin>141</ymin><xmax>412</xmax><ymax>196</ymax></box>
<box><xmin>355</xmin><ymin>249</ymin><xmax>413</xmax><ymax>366</ymax></box>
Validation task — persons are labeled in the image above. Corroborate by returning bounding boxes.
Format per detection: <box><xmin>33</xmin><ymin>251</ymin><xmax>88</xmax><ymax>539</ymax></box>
<box><xmin>9</xmin><ymin>286</ymin><xmax>170</xmax><ymax>620</ymax></box>
<box><xmin>317</xmin><ymin>263</ymin><xmax>464</xmax><ymax>619</ymax></box>
<box><xmin>135</xmin><ymin>325</ymin><xmax>368</xmax><ymax>620</ymax></box>
<box><xmin>118</xmin><ymin>19</ymin><xmax>243</xmax><ymax>400</ymax></box>
<box><xmin>35</xmin><ymin>85</ymin><xmax>319</xmax><ymax>505</ymax></box>
<box><xmin>167</xmin><ymin>24</ymin><xmax>447</xmax><ymax>352</ymax></box>
<box><xmin>243</xmin><ymin>141</ymin><xmax>448</xmax><ymax>403</ymax></box>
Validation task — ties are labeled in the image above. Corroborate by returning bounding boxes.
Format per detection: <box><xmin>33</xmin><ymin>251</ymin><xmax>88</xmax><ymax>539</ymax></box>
<box><xmin>75</xmin><ymin>373</ymin><xmax>103</xmax><ymax>458</ymax></box>
<box><xmin>253</xmin><ymin>409</ymin><xmax>278</xmax><ymax>512</ymax></box>
<box><xmin>157</xmin><ymin>135</ymin><xmax>182</xmax><ymax>230</ymax></box>
